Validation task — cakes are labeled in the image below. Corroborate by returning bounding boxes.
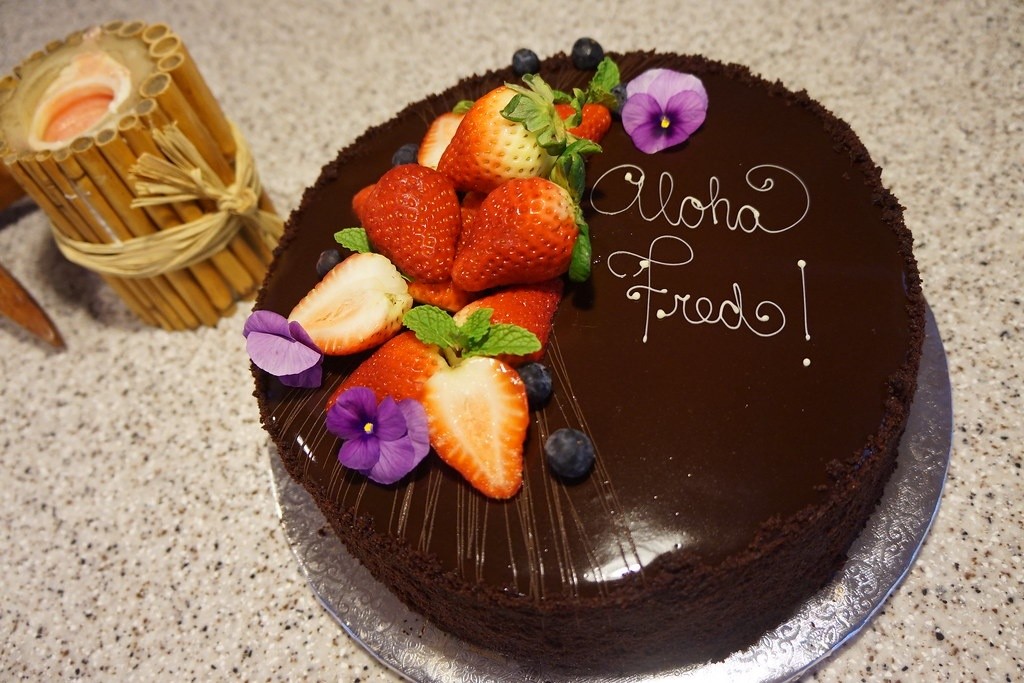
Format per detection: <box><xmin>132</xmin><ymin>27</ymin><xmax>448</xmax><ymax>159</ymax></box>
<box><xmin>244</xmin><ymin>35</ymin><xmax>926</xmax><ymax>676</ymax></box>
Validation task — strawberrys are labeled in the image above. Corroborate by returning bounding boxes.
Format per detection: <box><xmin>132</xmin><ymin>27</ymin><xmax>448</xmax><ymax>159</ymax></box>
<box><xmin>286</xmin><ymin>74</ymin><xmax>601</xmax><ymax>499</ymax></box>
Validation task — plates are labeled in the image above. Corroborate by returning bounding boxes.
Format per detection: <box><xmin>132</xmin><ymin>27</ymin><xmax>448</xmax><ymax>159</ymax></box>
<box><xmin>264</xmin><ymin>291</ymin><xmax>955</xmax><ymax>683</ymax></box>
